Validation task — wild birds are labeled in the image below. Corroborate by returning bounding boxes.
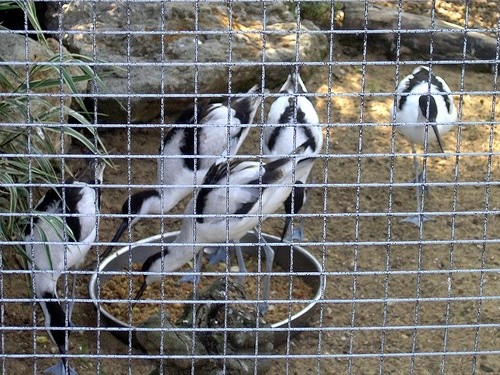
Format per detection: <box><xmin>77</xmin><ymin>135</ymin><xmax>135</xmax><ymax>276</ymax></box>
<box><xmin>392</xmin><ymin>66</ymin><xmax>457</xmax><ymax>229</ymax></box>
<box><xmin>262</xmin><ymin>71</ymin><xmax>323</xmax><ymax>243</ymax></box>
<box><xmin>20</xmin><ymin>148</ymin><xmax>115</xmax><ymax>375</ymax></box>
<box><xmin>96</xmin><ymin>76</ymin><xmax>270</xmax><ymax>285</ymax></box>
<box><xmin>132</xmin><ymin>136</ymin><xmax>317</xmax><ymax>317</ymax></box>
